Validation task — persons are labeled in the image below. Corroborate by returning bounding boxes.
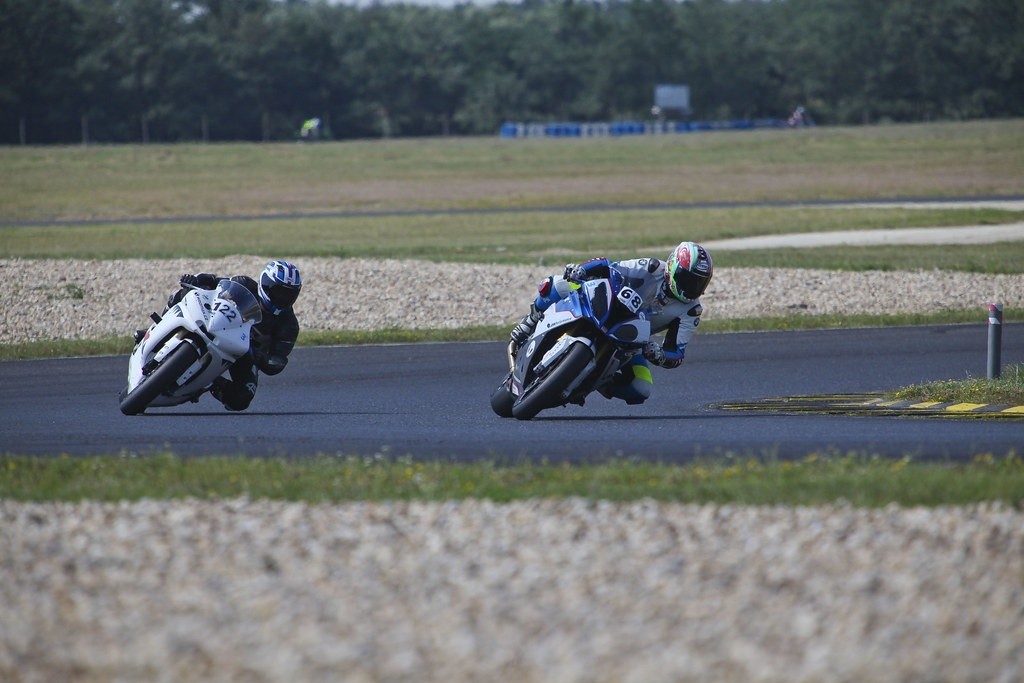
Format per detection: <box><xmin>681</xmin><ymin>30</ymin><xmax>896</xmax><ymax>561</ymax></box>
<box><xmin>510</xmin><ymin>240</ymin><xmax>712</xmax><ymax>404</ymax></box>
<box><xmin>135</xmin><ymin>259</ymin><xmax>303</xmax><ymax>412</ymax></box>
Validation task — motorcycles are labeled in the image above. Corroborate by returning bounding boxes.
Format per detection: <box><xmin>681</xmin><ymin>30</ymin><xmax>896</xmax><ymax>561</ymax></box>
<box><xmin>489</xmin><ymin>265</ymin><xmax>661</xmax><ymax>422</ymax></box>
<box><xmin>118</xmin><ymin>276</ymin><xmax>282</xmax><ymax>417</ymax></box>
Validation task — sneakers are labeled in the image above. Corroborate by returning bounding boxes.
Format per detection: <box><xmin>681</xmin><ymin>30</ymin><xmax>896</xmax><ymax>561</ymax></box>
<box><xmin>510</xmin><ymin>314</ymin><xmax>537</xmax><ymax>343</ymax></box>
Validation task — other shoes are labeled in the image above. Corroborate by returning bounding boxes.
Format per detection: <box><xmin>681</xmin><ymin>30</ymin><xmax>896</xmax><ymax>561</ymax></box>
<box><xmin>133</xmin><ymin>329</ymin><xmax>147</xmax><ymax>341</ymax></box>
<box><xmin>206</xmin><ymin>374</ymin><xmax>231</xmax><ymax>402</ymax></box>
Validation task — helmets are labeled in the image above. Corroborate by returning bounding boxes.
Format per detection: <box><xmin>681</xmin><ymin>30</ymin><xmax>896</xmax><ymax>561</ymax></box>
<box><xmin>663</xmin><ymin>241</ymin><xmax>713</xmax><ymax>304</ymax></box>
<box><xmin>258</xmin><ymin>259</ymin><xmax>302</xmax><ymax>316</ymax></box>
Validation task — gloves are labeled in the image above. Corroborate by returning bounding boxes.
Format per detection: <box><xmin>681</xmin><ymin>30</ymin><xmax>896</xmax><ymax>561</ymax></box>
<box><xmin>642</xmin><ymin>340</ymin><xmax>666</xmax><ymax>366</ymax></box>
<box><xmin>250</xmin><ymin>351</ymin><xmax>269</xmax><ymax>367</ymax></box>
<box><xmin>179</xmin><ymin>271</ymin><xmax>216</xmax><ymax>292</ymax></box>
<box><xmin>562</xmin><ymin>262</ymin><xmax>587</xmax><ymax>284</ymax></box>
